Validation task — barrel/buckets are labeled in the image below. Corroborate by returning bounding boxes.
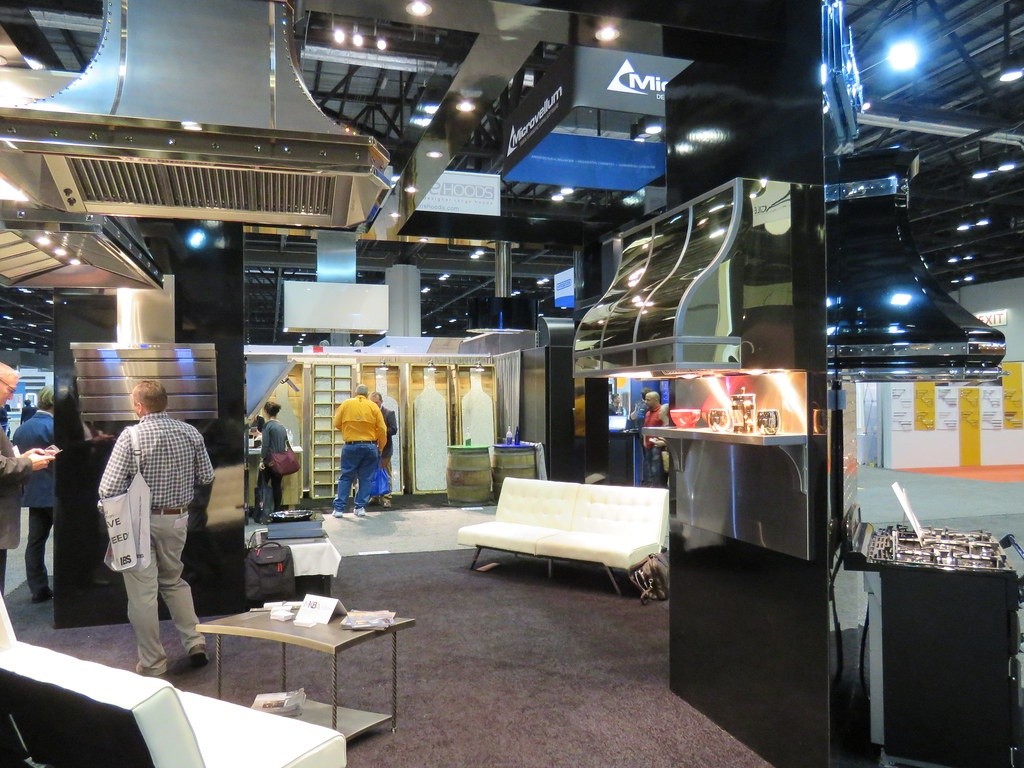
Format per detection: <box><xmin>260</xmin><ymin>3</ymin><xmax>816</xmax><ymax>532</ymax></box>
<box><xmin>445</xmin><ymin>445</ymin><xmax>492</xmax><ymax>506</ymax></box>
<box><xmin>493</xmin><ymin>444</ymin><xmax>536</xmax><ymax>501</ymax></box>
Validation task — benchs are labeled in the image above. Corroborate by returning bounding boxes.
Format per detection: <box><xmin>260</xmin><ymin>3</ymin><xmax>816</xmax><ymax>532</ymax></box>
<box><xmin>456</xmin><ymin>476</ymin><xmax>669</xmax><ymax>598</ymax></box>
<box><xmin>0</xmin><ymin>642</ymin><xmax>348</xmax><ymax>768</ymax></box>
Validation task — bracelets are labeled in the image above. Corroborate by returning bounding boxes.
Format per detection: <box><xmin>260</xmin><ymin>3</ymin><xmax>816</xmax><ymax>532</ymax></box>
<box><xmin>260</xmin><ymin>461</ymin><xmax>264</xmax><ymax>462</ymax></box>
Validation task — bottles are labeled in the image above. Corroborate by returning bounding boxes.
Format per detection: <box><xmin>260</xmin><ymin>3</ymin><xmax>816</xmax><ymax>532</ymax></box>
<box><xmin>731</xmin><ymin>388</ymin><xmax>756</xmax><ymax>433</ymax></box>
<box><xmin>515</xmin><ymin>427</ymin><xmax>520</xmax><ymax>445</ymax></box>
<box><xmin>506</xmin><ymin>426</ymin><xmax>513</xmax><ymax>445</ymax></box>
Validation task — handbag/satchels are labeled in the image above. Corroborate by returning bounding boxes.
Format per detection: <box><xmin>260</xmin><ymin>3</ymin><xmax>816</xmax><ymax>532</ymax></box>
<box><xmin>626</xmin><ymin>553</ymin><xmax>672</xmax><ymax>605</ymax></box>
<box><xmin>661</xmin><ymin>450</ymin><xmax>671</xmax><ymax>474</ymax></box>
<box><xmin>368</xmin><ymin>453</ymin><xmax>391</xmax><ymax>497</ymax></box>
<box><xmin>240</xmin><ymin>531</ymin><xmax>297</xmax><ymax>603</ymax></box>
<box><xmin>97</xmin><ymin>426</ymin><xmax>152</xmax><ymax>574</ymax></box>
<box><xmin>265</xmin><ymin>448</ymin><xmax>301</xmax><ymax>477</ymax></box>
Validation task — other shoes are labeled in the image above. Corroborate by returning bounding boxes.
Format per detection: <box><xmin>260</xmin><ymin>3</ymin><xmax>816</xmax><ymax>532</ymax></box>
<box><xmin>353</xmin><ymin>507</ymin><xmax>366</xmax><ymax>516</ymax></box>
<box><xmin>380</xmin><ymin>494</ymin><xmax>392</xmax><ymax>508</ymax></box>
<box><xmin>367</xmin><ymin>496</ymin><xmax>379</xmax><ymax>506</ymax></box>
<box><xmin>135</xmin><ymin>659</ymin><xmax>167</xmax><ymax>676</ymax></box>
<box><xmin>31</xmin><ymin>588</ymin><xmax>54</xmax><ymax>603</ymax></box>
<box><xmin>331</xmin><ymin>509</ymin><xmax>343</xmax><ymax>518</ymax></box>
<box><xmin>188</xmin><ymin>644</ymin><xmax>210</xmax><ymax>668</ymax></box>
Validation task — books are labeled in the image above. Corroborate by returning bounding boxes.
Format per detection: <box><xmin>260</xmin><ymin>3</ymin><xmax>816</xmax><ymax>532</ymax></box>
<box><xmin>340</xmin><ymin>610</ymin><xmax>396</xmax><ymax>631</ymax></box>
<box><xmin>251</xmin><ymin>688</ymin><xmax>306</xmax><ymax>716</ymax></box>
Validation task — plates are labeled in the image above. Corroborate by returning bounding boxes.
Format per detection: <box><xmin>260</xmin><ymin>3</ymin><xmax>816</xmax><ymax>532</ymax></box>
<box><xmin>268</xmin><ymin>509</ymin><xmax>313</xmax><ymax>521</ymax></box>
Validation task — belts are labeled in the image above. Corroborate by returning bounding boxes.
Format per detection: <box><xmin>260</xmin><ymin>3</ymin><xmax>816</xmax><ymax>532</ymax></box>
<box><xmin>344</xmin><ymin>440</ymin><xmax>377</xmax><ymax>444</ymax></box>
<box><xmin>151</xmin><ymin>507</ymin><xmax>188</xmax><ymax>514</ymax></box>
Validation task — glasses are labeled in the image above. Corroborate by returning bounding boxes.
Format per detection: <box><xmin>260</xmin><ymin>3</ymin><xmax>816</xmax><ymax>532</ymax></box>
<box><xmin>1</xmin><ymin>379</ymin><xmax>16</xmax><ymax>395</ymax></box>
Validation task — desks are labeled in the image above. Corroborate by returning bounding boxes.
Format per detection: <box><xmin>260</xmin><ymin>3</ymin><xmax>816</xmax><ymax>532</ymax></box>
<box><xmin>253</xmin><ymin>528</ymin><xmax>342</xmax><ymax>601</ymax></box>
<box><xmin>195</xmin><ymin>612</ymin><xmax>416</xmax><ymax>741</ymax></box>
<box><xmin>248</xmin><ymin>447</ymin><xmax>304</xmax><ymax>506</ymax></box>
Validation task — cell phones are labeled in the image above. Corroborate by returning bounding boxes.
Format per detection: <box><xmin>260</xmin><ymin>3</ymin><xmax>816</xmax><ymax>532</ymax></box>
<box><xmin>52</xmin><ymin>449</ymin><xmax>63</xmax><ymax>457</ymax></box>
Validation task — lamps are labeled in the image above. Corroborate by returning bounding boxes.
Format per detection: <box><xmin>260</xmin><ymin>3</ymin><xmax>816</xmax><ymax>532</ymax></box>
<box><xmin>630</xmin><ymin>114</ymin><xmax>662</xmax><ymax>141</ymax></box>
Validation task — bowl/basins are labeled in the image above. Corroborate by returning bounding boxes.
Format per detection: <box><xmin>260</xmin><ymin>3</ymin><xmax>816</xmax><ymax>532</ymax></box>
<box><xmin>670</xmin><ymin>409</ymin><xmax>702</xmax><ymax>429</ymax></box>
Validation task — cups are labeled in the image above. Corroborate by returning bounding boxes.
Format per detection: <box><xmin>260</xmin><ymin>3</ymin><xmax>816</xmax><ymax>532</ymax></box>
<box><xmin>707</xmin><ymin>409</ymin><xmax>729</xmax><ymax>432</ymax></box>
<box><xmin>758</xmin><ymin>410</ymin><xmax>779</xmax><ymax>436</ymax></box>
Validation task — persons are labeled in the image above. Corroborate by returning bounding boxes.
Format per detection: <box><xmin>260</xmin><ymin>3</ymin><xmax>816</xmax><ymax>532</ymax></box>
<box><xmin>252</xmin><ymin>401</ymin><xmax>287</xmax><ymax>524</ymax></box>
<box><xmin>575</xmin><ymin>386</ymin><xmax>585</xmax><ymax>480</ymax></box>
<box><xmin>609</xmin><ymin>393</ymin><xmax>623</xmax><ymax>415</ymax></box>
<box><xmin>0</xmin><ymin>405</ymin><xmax>11</xmax><ymax>439</ymax></box>
<box><xmin>332</xmin><ymin>384</ymin><xmax>387</xmax><ymax>517</ymax></box>
<box><xmin>14</xmin><ymin>386</ymin><xmax>55</xmax><ymax>602</ymax></box>
<box><xmin>0</xmin><ymin>361</ymin><xmax>55</xmax><ymax>596</ymax></box>
<box><xmin>97</xmin><ymin>381</ymin><xmax>215</xmax><ymax>676</ymax></box>
<box><xmin>624</xmin><ymin>389</ymin><xmax>669</xmax><ymax>486</ymax></box>
<box><xmin>20</xmin><ymin>400</ymin><xmax>37</xmax><ymax>425</ymax></box>
<box><xmin>369</xmin><ymin>392</ymin><xmax>397</xmax><ymax>507</ymax></box>
<box><xmin>249</xmin><ymin>415</ymin><xmax>265</xmax><ymax>439</ymax></box>
